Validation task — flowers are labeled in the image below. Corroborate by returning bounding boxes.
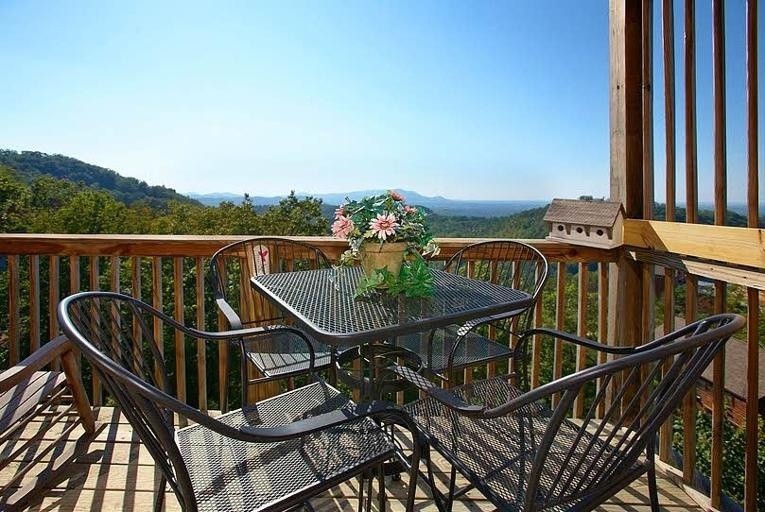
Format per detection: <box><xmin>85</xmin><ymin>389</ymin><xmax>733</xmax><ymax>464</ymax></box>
<box><xmin>331</xmin><ymin>189</ymin><xmax>442</xmax><ymax>300</ymax></box>
